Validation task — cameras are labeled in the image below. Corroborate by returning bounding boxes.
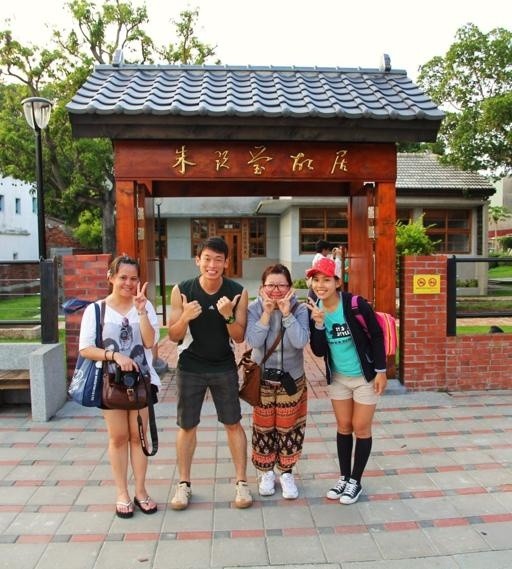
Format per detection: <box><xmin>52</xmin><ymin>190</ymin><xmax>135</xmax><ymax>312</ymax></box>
<box><xmin>263</xmin><ymin>368</ymin><xmax>285</xmax><ymax>382</ymax></box>
<box><xmin>114</xmin><ymin>371</ymin><xmax>140</xmax><ymax>388</ymax></box>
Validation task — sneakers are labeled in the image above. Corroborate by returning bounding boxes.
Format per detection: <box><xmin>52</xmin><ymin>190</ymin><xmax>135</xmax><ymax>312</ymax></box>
<box><xmin>231</xmin><ymin>478</ymin><xmax>254</xmax><ymax>509</ymax></box>
<box><xmin>339</xmin><ymin>477</ymin><xmax>363</xmax><ymax>504</ymax></box>
<box><xmin>326</xmin><ymin>478</ymin><xmax>350</xmax><ymax>500</ymax></box>
<box><xmin>278</xmin><ymin>472</ymin><xmax>298</xmax><ymax>500</ymax></box>
<box><xmin>256</xmin><ymin>471</ymin><xmax>277</xmax><ymax>497</ymax></box>
<box><xmin>168</xmin><ymin>480</ymin><xmax>193</xmax><ymax>512</ymax></box>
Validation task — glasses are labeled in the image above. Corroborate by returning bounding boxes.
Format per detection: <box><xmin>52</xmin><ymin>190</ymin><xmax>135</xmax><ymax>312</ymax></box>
<box><xmin>263</xmin><ymin>282</ymin><xmax>291</xmax><ymax>291</ymax></box>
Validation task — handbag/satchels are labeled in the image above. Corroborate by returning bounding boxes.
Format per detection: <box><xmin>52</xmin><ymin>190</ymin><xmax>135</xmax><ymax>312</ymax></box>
<box><xmin>68</xmin><ymin>301</ymin><xmax>102</xmax><ymax>408</ymax></box>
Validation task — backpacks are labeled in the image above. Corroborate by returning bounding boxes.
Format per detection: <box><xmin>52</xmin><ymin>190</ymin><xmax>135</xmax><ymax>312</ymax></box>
<box><xmin>373</xmin><ymin>311</ymin><xmax>397</xmax><ymax>357</ymax></box>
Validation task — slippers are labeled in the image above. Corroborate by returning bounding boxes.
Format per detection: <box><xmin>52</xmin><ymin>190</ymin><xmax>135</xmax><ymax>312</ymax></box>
<box><xmin>133</xmin><ymin>493</ymin><xmax>158</xmax><ymax>514</ymax></box>
<box><xmin>115</xmin><ymin>497</ymin><xmax>135</xmax><ymax>518</ymax></box>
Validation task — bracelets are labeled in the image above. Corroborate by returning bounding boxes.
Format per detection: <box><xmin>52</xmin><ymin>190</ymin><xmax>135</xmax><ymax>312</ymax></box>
<box><xmin>111</xmin><ymin>350</ymin><xmax>115</xmax><ymax>362</ymax></box>
<box><xmin>104</xmin><ymin>349</ymin><xmax>109</xmax><ymax>362</ymax></box>
<box><xmin>138</xmin><ymin>310</ymin><xmax>148</xmax><ymax>315</ymax></box>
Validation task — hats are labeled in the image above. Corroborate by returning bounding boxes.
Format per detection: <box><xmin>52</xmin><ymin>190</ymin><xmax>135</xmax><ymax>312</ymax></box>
<box><xmin>303</xmin><ymin>252</ymin><xmax>342</xmax><ymax>282</ymax></box>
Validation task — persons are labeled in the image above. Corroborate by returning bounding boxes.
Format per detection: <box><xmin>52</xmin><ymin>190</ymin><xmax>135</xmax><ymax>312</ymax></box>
<box><xmin>327</xmin><ymin>248</ymin><xmax>343</xmax><ymax>291</ymax></box>
<box><xmin>339</xmin><ymin>244</ymin><xmax>349</xmax><ymax>293</ymax></box>
<box><xmin>302</xmin><ymin>258</ymin><xmax>388</xmax><ymax>507</ymax></box>
<box><xmin>167</xmin><ymin>235</ymin><xmax>253</xmax><ymax>511</ymax></box>
<box><xmin>306</xmin><ymin>240</ymin><xmax>330</xmax><ymax>304</ymax></box>
<box><xmin>79</xmin><ymin>255</ymin><xmax>161</xmax><ymax>519</ymax></box>
<box><xmin>245</xmin><ymin>264</ymin><xmax>310</xmax><ymax>500</ymax></box>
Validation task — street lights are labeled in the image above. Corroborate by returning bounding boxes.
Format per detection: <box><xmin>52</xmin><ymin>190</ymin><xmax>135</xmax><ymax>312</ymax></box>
<box><xmin>20</xmin><ymin>94</ymin><xmax>55</xmax><ymax>261</ymax></box>
<box><xmin>154</xmin><ymin>195</ymin><xmax>168</xmax><ymax>329</ymax></box>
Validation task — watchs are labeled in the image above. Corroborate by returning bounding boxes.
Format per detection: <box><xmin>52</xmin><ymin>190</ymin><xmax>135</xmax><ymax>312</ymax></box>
<box><xmin>223</xmin><ymin>315</ymin><xmax>236</xmax><ymax>325</ymax></box>
